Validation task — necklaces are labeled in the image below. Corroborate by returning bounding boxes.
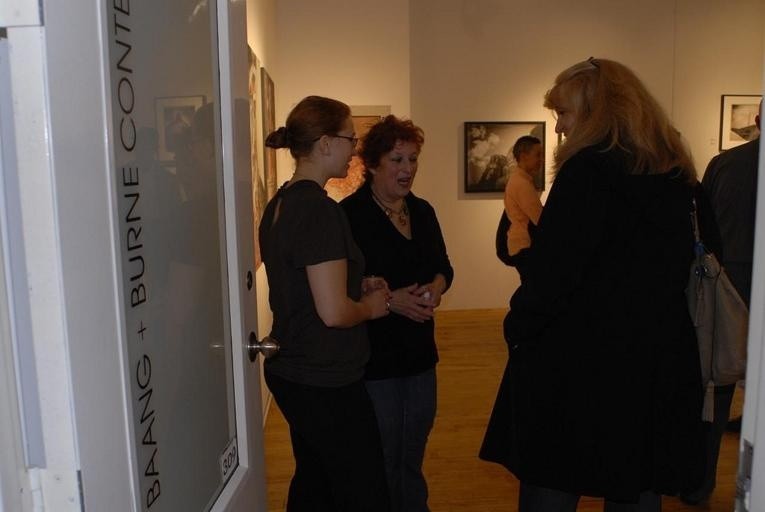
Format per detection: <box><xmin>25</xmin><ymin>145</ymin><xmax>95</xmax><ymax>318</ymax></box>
<box><xmin>374</xmin><ymin>193</ymin><xmax>409</xmax><ymax>225</ymax></box>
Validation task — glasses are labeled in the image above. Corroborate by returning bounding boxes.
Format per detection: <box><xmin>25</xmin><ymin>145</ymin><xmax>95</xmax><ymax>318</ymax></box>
<box><xmin>333</xmin><ymin>135</ymin><xmax>358</xmax><ymax>147</ymax></box>
<box><xmin>588</xmin><ymin>57</ymin><xmax>600</xmax><ymax>67</ymax></box>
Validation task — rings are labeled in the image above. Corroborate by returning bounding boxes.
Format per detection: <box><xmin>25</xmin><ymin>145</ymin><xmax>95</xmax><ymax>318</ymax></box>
<box><xmin>386</xmin><ymin>302</ymin><xmax>391</xmax><ymax>308</ymax></box>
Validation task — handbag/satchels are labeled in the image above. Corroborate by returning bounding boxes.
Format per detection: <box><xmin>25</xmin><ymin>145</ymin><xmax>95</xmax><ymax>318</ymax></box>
<box><xmin>687</xmin><ymin>197</ymin><xmax>749</xmax><ymax>387</ymax></box>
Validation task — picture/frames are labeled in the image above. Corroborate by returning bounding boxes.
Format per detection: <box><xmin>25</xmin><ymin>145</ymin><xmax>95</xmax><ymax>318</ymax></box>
<box><xmin>719</xmin><ymin>94</ymin><xmax>765</xmax><ymax>151</ymax></box>
<box><xmin>464</xmin><ymin>120</ymin><xmax>547</xmax><ymax>194</ymax></box>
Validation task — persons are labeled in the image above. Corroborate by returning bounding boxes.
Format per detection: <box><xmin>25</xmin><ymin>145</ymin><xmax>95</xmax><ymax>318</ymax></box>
<box><xmin>475</xmin><ymin>56</ymin><xmax>707</xmax><ymax>510</ymax></box>
<box><xmin>261</xmin><ymin>95</ymin><xmax>394</xmax><ymax>512</ymax></box>
<box><xmin>500</xmin><ymin>135</ymin><xmax>544</xmax><ymax>284</ymax></box>
<box><xmin>337</xmin><ymin>114</ymin><xmax>454</xmax><ymax>511</ymax></box>
<box><xmin>675</xmin><ymin>95</ymin><xmax>763</xmax><ymax>507</ymax></box>
